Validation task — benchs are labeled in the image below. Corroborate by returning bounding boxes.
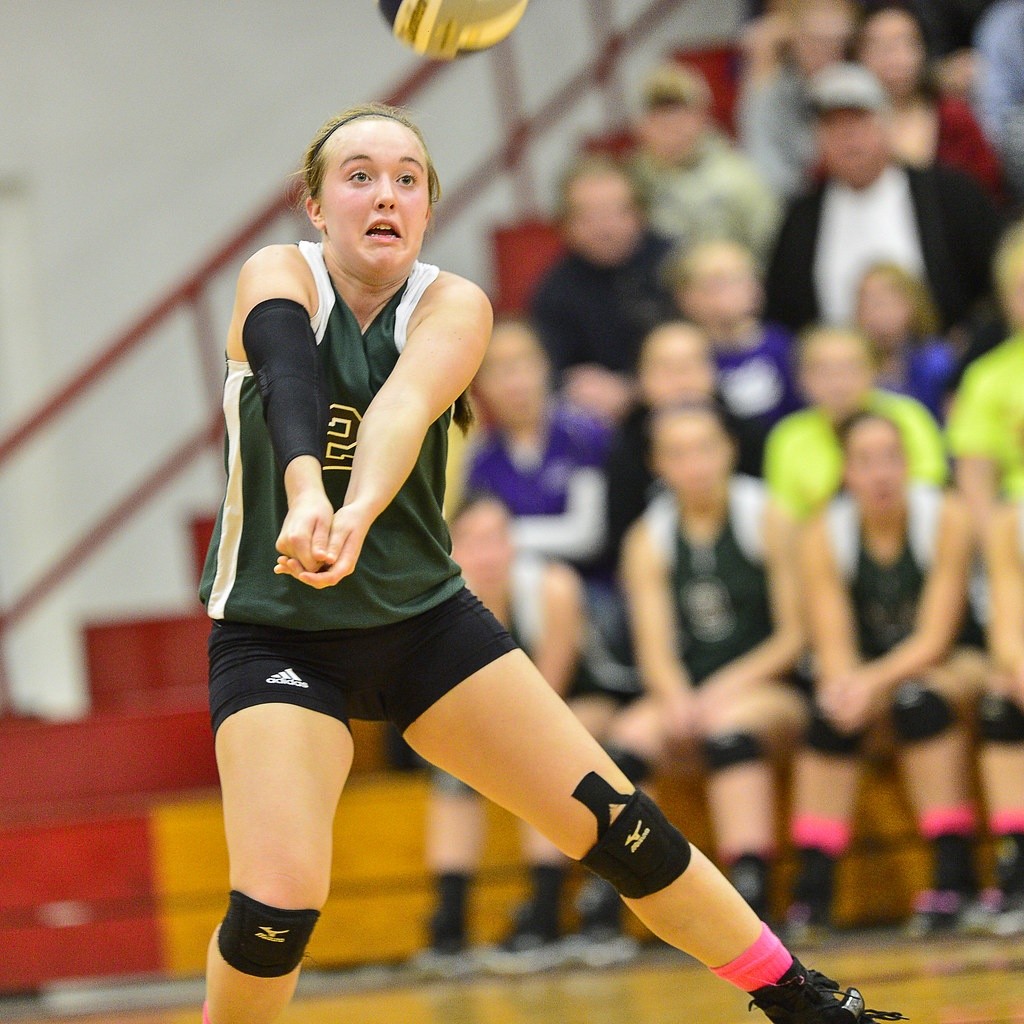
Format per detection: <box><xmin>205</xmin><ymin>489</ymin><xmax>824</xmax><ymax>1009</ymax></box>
<box><xmin>0</xmin><ymin>45</ymin><xmax>990</xmax><ymax>990</ymax></box>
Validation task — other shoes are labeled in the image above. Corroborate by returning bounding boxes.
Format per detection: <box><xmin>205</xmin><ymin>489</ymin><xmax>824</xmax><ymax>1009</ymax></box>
<box><xmin>480</xmin><ymin>925</ymin><xmax>575</xmax><ymax>973</ymax></box>
<box><xmin>959</xmin><ymin>900</ymin><xmax>1024</xmax><ymax>939</ymax></box>
<box><xmin>572</xmin><ymin>925</ymin><xmax>640</xmax><ymax>967</ymax></box>
<box><xmin>413</xmin><ymin>931</ymin><xmax>478</xmax><ymax>979</ymax></box>
<box><xmin>897</xmin><ymin>893</ymin><xmax>956</xmax><ymax>940</ymax></box>
<box><xmin>749</xmin><ymin>956</ymin><xmax>909</xmax><ymax>1024</ymax></box>
<box><xmin>787</xmin><ymin>916</ymin><xmax>847</xmax><ymax>948</ymax></box>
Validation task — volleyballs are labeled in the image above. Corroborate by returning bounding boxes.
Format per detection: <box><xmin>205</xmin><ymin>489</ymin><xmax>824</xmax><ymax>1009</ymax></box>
<box><xmin>378</xmin><ymin>1</ymin><xmax>529</xmax><ymax>59</ymax></box>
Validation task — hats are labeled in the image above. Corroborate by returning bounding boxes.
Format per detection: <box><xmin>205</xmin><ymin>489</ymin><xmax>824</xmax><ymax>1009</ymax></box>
<box><xmin>805</xmin><ymin>64</ymin><xmax>889</xmax><ymax>115</ymax></box>
<box><xmin>644</xmin><ymin>67</ymin><xmax>705</xmax><ymax>108</ymax></box>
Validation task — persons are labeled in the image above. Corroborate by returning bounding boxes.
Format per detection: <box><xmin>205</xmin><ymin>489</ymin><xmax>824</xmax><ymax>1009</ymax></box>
<box><xmin>202</xmin><ymin>108</ymin><xmax>901</xmax><ymax>1024</ymax></box>
<box><xmin>409</xmin><ymin>0</ymin><xmax>1024</xmax><ymax>979</ymax></box>
